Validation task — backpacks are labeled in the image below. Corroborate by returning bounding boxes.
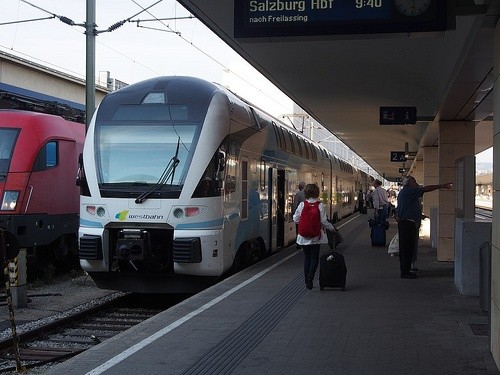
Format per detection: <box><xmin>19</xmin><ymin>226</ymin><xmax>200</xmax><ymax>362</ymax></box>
<box><xmin>298</xmin><ymin>201</ymin><xmax>321</xmax><ymax>237</ymax></box>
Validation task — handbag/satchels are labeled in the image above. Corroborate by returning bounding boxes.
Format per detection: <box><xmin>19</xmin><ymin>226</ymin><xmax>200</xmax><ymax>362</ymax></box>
<box><xmin>326</xmin><ymin>227</ymin><xmax>344</xmax><ymax>248</ymax></box>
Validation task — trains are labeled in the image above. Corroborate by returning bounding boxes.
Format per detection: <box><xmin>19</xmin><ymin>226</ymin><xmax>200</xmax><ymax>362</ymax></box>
<box><xmin>73</xmin><ymin>74</ymin><xmax>377</xmax><ymax>295</ymax></box>
<box><xmin>0</xmin><ymin>107</ymin><xmax>86</xmax><ymax>282</ymax></box>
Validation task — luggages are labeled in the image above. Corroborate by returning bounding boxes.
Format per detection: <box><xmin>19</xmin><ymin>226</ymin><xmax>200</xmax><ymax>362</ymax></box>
<box><xmin>368</xmin><ymin>215</ymin><xmax>391</xmax><ymax>248</ymax></box>
<box><xmin>362</xmin><ymin>207</ymin><xmax>367</xmax><ymax>214</ymax></box>
<box><xmin>319</xmin><ymin>229</ymin><xmax>347</xmax><ymax>291</ymax></box>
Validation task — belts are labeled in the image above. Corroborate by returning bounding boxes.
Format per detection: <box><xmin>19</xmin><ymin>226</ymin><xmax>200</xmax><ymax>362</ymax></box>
<box><xmin>400</xmin><ymin>218</ymin><xmax>416</xmax><ymax>223</ymax></box>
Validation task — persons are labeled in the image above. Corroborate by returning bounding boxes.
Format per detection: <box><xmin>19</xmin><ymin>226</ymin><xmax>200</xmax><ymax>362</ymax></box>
<box><xmin>249</xmin><ymin>182</ymin><xmax>264</xmax><ymax>234</ymax></box>
<box><xmin>373</xmin><ymin>179</ymin><xmax>388</xmax><ymax>224</ymax></box>
<box><xmin>397</xmin><ymin>176</ymin><xmax>454</xmax><ymax>280</ymax></box>
<box><xmin>293</xmin><ymin>184</ymin><xmax>335</xmax><ymax>289</ymax></box>
<box><xmin>291</xmin><ymin>181</ymin><xmax>306</xmax><ymax>250</ymax></box>
<box><xmin>387</xmin><ymin>188</ymin><xmax>397</xmax><ymax>217</ymax></box>
<box><xmin>355</xmin><ymin>188</ymin><xmax>374</xmax><ymax>214</ymax></box>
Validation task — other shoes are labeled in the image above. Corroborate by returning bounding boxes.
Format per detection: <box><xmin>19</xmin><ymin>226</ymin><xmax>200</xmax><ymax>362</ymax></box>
<box><xmin>305</xmin><ymin>272</ymin><xmax>314</xmax><ymax>290</ymax></box>
<box><xmin>392</xmin><ymin>215</ymin><xmax>395</xmax><ymax>217</ymax></box>
<box><xmin>401</xmin><ymin>272</ymin><xmax>417</xmax><ymax>279</ymax></box>
<box><xmin>410</xmin><ymin>267</ymin><xmax>421</xmax><ymax>273</ymax></box>
<box><xmin>296</xmin><ymin>244</ymin><xmax>301</xmax><ymax>249</ymax></box>
